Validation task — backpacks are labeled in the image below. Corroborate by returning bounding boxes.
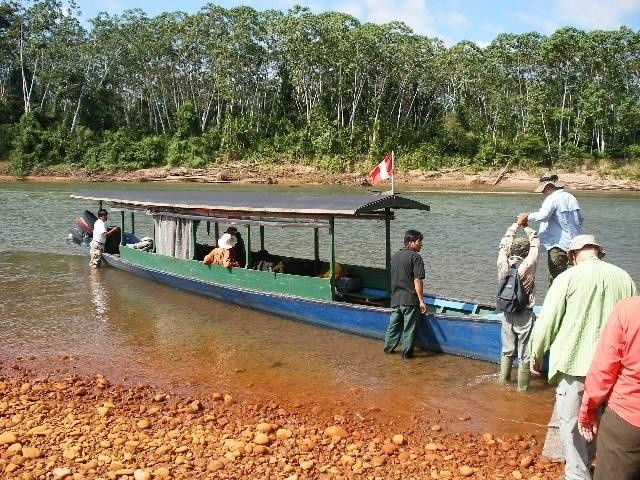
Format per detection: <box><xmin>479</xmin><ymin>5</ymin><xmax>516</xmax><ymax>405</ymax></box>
<box><xmin>496</xmin><ymin>258</ymin><xmax>527</xmax><ymax>313</ymax></box>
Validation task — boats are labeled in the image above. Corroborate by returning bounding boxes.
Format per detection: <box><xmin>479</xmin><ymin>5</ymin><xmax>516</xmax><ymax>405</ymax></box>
<box><xmin>68</xmin><ymin>195</ymin><xmax>550</xmax><ymax>372</ymax></box>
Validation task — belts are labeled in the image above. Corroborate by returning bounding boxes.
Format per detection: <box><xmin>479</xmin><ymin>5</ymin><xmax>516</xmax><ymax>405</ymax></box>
<box><xmin>93</xmin><ymin>239</ymin><xmax>103</xmax><ymax>245</ymax></box>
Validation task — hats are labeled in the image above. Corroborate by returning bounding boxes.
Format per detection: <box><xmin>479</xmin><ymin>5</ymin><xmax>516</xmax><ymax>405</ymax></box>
<box><xmin>511</xmin><ymin>238</ymin><xmax>530</xmax><ymax>251</ymax></box>
<box><xmin>218</xmin><ymin>227</ymin><xmax>237</xmax><ymax>249</ymax></box>
<box><xmin>534</xmin><ymin>172</ymin><xmax>564</xmax><ymax>192</ymax></box>
<box><xmin>567</xmin><ymin>234</ymin><xmax>606</xmax><ymax>265</ymax></box>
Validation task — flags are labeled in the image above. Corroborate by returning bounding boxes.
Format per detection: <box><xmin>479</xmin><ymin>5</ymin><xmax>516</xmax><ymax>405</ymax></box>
<box><xmin>368</xmin><ymin>155</ymin><xmax>392</xmax><ymax>185</ymax></box>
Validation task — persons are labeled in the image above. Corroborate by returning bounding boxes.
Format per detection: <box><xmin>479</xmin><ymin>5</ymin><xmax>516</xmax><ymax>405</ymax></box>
<box><xmin>88</xmin><ymin>209</ymin><xmax>119</xmax><ymax>269</ymax></box>
<box><xmin>384</xmin><ymin>230</ymin><xmax>427</xmax><ymax>358</ymax></box>
<box><xmin>203</xmin><ymin>227</ymin><xmax>246</xmax><ymax>268</ymax></box>
<box><xmin>576</xmin><ymin>294</ymin><xmax>640</xmax><ymax>479</ymax></box>
<box><xmin>529</xmin><ymin>236</ymin><xmax>637</xmax><ymax>479</ymax></box>
<box><xmin>520</xmin><ymin>173</ymin><xmax>585</xmax><ymax>290</ymax></box>
<box><xmin>497</xmin><ymin>213</ymin><xmax>541</xmax><ymax>393</ymax></box>
